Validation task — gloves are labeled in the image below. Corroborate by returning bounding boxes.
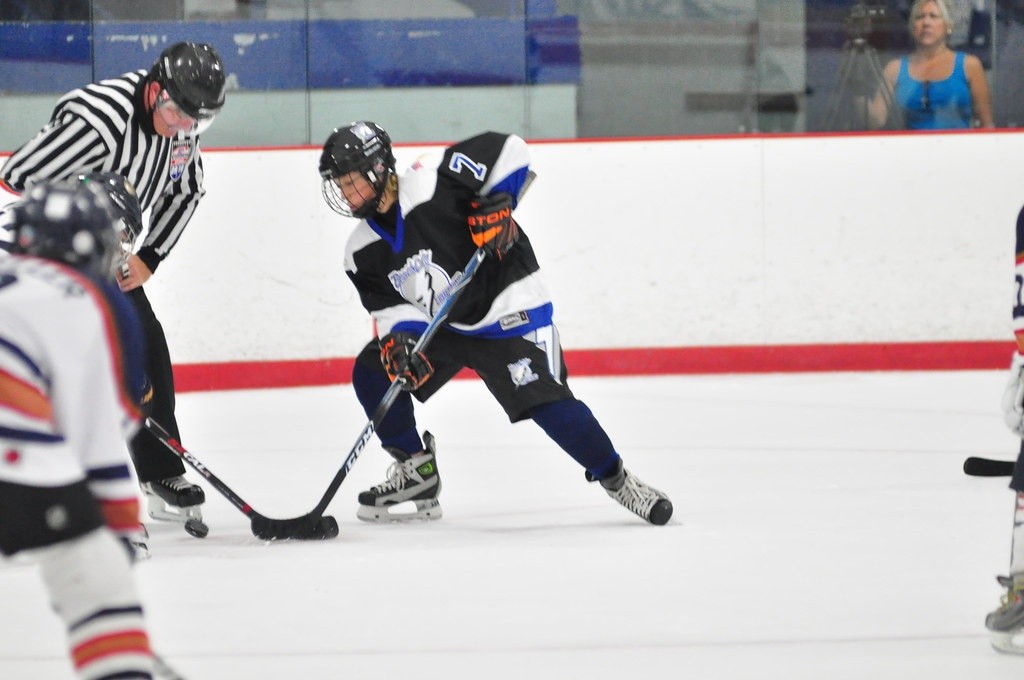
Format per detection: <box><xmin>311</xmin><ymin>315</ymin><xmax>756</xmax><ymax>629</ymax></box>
<box><xmin>377</xmin><ymin>333</ymin><xmax>434</xmax><ymax>391</ymax></box>
<box><xmin>467</xmin><ymin>193</ymin><xmax>514</xmax><ymax>254</ymax></box>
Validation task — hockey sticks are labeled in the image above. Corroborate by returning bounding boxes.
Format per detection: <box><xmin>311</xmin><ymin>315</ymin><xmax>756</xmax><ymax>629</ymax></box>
<box><xmin>962</xmin><ymin>456</ymin><xmax>1016</xmax><ymax>480</ymax></box>
<box><xmin>139</xmin><ymin>414</ymin><xmax>296</xmax><ymax>539</ymax></box>
<box><xmin>298</xmin><ymin>168</ymin><xmax>537</xmax><ymax>544</ymax></box>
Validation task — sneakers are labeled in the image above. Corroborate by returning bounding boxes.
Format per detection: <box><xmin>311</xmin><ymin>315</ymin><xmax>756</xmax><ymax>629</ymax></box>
<box><xmin>139</xmin><ymin>475</ymin><xmax>205</xmax><ymax>524</ymax></box>
<box><xmin>986</xmin><ymin>575</ymin><xmax>1024</xmax><ymax>654</ymax></box>
<box><xmin>358</xmin><ymin>431</ymin><xmax>445</xmax><ymax>524</ymax></box>
<box><xmin>602</xmin><ymin>471</ymin><xmax>681</xmax><ymax>525</ymax></box>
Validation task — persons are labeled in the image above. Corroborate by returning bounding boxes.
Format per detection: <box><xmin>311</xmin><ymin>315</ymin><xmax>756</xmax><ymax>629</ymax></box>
<box><xmin>868</xmin><ymin>0</ymin><xmax>997</xmax><ymax>131</ymax></box>
<box><xmin>0</xmin><ymin>172</ymin><xmax>184</xmax><ymax>680</ymax></box>
<box><xmin>0</xmin><ymin>42</ymin><xmax>228</xmax><ymax>537</ymax></box>
<box><xmin>318</xmin><ymin>121</ymin><xmax>674</xmax><ymax>526</ymax></box>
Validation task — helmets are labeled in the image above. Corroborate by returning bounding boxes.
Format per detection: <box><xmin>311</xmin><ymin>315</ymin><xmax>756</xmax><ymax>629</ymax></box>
<box><xmin>319</xmin><ymin>121</ymin><xmax>392</xmax><ymax>218</ymax></box>
<box><xmin>89</xmin><ymin>174</ymin><xmax>143</xmax><ymax>246</ymax></box>
<box><xmin>151</xmin><ymin>41</ymin><xmax>224</xmax><ymax>119</ymax></box>
<box><xmin>13</xmin><ymin>175</ymin><xmax>114</xmax><ymax>282</ymax></box>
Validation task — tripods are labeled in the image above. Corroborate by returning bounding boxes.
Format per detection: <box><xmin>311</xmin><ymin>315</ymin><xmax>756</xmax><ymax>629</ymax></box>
<box><xmin>823</xmin><ymin>38</ymin><xmax>908</xmax><ymax>133</ymax></box>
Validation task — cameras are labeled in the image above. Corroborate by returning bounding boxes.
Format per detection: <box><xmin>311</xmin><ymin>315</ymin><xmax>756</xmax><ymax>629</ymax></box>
<box><xmin>846</xmin><ymin>3</ymin><xmax>887</xmax><ymax>39</ymax></box>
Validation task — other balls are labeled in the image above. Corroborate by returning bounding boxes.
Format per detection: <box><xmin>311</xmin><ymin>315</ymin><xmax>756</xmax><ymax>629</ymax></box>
<box><xmin>183</xmin><ymin>517</ymin><xmax>210</xmax><ymax>539</ymax></box>
